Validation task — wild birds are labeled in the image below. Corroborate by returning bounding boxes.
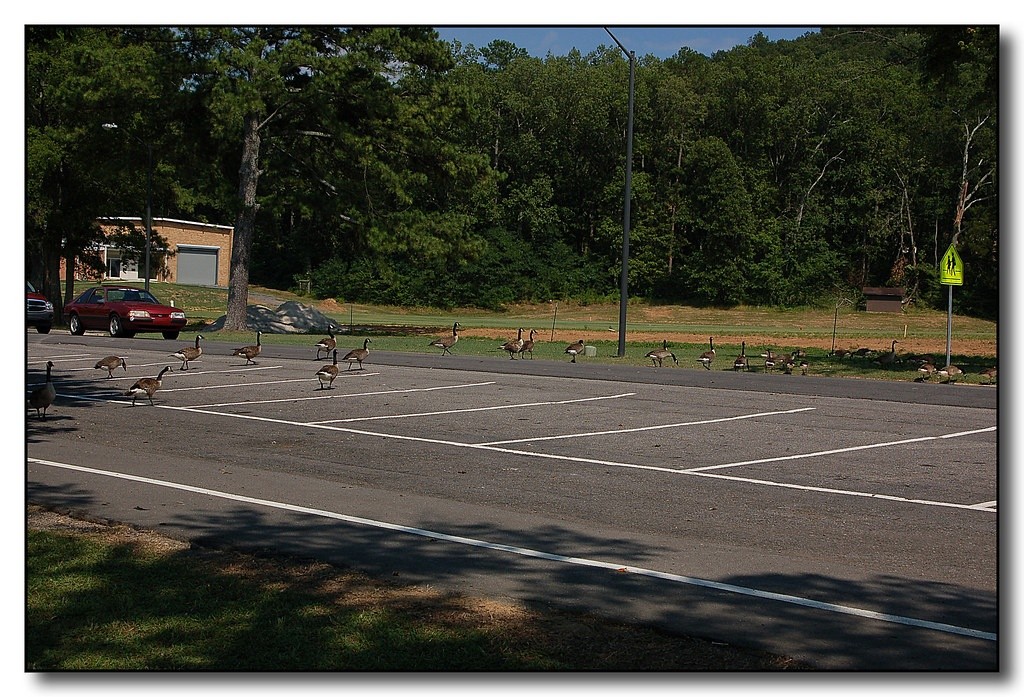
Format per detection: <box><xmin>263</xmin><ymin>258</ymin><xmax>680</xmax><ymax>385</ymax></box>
<box><xmin>644</xmin><ymin>337</ymin><xmax>997</xmax><ymax>385</ymax></box>
<box><xmin>230</xmin><ymin>330</ymin><xmax>262</xmax><ymax>366</ymax></box>
<box><xmin>497</xmin><ymin>327</ymin><xmax>537</xmax><ymax>360</ymax></box>
<box><xmin>125</xmin><ymin>366</ymin><xmax>173</xmax><ymax>407</ymax></box>
<box><xmin>564</xmin><ymin>340</ymin><xmax>585</xmax><ymax>363</ymax></box>
<box><xmin>427</xmin><ymin>322</ymin><xmax>462</xmax><ymax>356</ymax></box>
<box><xmin>167</xmin><ymin>334</ymin><xmax>205</xmax><ymax>371</ymax></box>
<box><xmin>93</xmin><ymin>356</ymin><xmax>126</xmax><ymax>379</ymax></box>
<box><xmin>314</xmin><ymin>324</ymin><xmax>372</xmax><ymax>390</ymax></box>
<box><xmin>28</xmin><ymin>361</ymin><xmax>56</xmax><ymax>422</ymax></box>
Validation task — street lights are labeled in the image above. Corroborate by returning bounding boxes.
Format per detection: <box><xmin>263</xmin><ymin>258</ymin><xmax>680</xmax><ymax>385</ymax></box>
<box><xmin>102</xmin><ymin>121</ymin><xmax>153</xmax><ymax>300</ymax></box>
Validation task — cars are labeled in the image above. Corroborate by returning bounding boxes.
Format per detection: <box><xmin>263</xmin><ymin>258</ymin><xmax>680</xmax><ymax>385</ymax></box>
<box><xmin>62</xmin><ymin>283</ymin><xmax>187</xmax><ymax>340</ymax></box>
<box><xmin>26</xmin><ymin>280</ymin><xmax>55</xmax><ymax>335</ymax></box>
